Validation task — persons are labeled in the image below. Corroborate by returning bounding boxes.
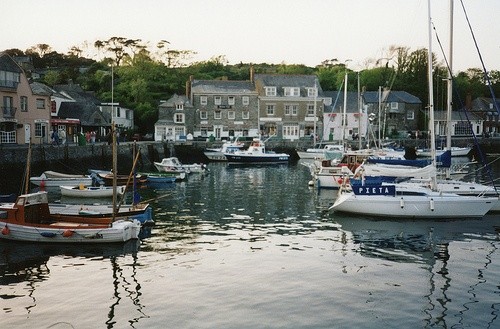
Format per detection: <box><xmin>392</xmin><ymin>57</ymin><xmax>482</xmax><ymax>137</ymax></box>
<box><xmin>85</xmin><ymin>130</ymin><xmax>97</xmax><ymax>144</ymax></box>
<box><xmin>49</xmin><ymin>129</ymin><xmax>68</xmax><ymax>145</ymax></box>
<box><xmin>107</xmin><ymin>128</ymin><xmax>127</xmax><ymax>145</ymax></box>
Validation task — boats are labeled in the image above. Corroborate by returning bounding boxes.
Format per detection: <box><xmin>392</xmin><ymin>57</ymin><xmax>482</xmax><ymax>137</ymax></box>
<box><xmin>0</xmin><ymin>140</ymin><xmax>154</xmax><ymax>225</ymax></box>
<box><xmin>153</xmin><ymin>156</ymin><xmax>211</xmax><ymax>174</ymax></box>
<box><xmin>223</xmin><ymin>139</ymin><xmax>290</xmax><ymax>165</ymax></box>
<box><xmin>203</xmin><ymin>136</ymin><xmax>276</xmax><ymax>161</ymax></box>
<box><xmin>59</xmin><ymin>183</ymin><xmax>127</xmax><ymax>197</ymax></box>
<box><xmin>29</xmin><ymin>168</ymin><xmax>186</xmax><ymax>187</ymax></box>
<box><xmin>0</xmin><ymin>65</ymin><xmax>142</xmax><ymax>245</ymax></box>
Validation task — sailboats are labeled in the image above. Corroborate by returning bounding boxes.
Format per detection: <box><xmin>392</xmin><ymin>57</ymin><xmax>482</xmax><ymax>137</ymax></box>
<box><xmin>325</xmin><ymin>0</ymin><xmax>500</xmax><ymax>219</ymax></box>
<box><xmin>294</xmin><ymin>0</ymin><xmax>500</xmax><ymax>213</ymax></box>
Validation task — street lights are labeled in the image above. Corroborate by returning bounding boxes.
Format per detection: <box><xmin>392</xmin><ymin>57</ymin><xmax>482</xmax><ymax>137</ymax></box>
<box><xmin>108</xmin><ymin>61</ymin><xmax>117</xmax><ymax>122</ymax></box>
